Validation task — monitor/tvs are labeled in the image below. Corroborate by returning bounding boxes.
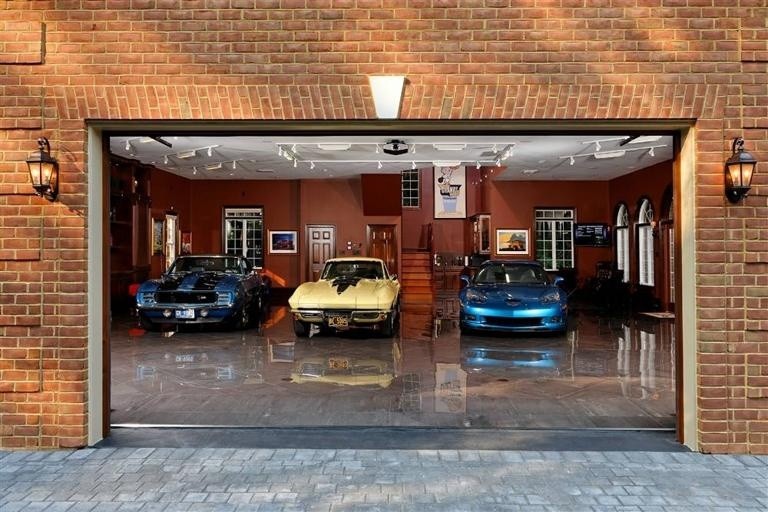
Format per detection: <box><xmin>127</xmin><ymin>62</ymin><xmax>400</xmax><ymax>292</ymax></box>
<box><xmin>573</xmin><ymin>222</ymin><xmax>610</xmax><ymax>248</ymax></box>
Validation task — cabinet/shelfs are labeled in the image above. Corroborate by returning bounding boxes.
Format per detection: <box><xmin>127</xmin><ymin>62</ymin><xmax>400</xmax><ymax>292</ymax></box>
<box><xmin>469</xmin><ymin>212</ymin><xmax>492</xmax><ymax>258</ymax></box>
<box><xmin>110</xmin><ymin>157</ymin><xmax>151</xmax><ymax>312</ymax></box>
<box><xmin>435</xmin><ymin>267</ymin><xmax>469</xmax><ymax>296</ymax></box>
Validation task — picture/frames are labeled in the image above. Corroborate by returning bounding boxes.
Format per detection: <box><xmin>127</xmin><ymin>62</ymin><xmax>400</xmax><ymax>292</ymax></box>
<box><xmin>432</xmin><ymin>166</ymin><xmax>468</xmax><ymax>221</ymax></box>
<box><xmin>152</xmin><ymin>217</ymin><xmax>167</xmax><ymax>257</ymax></box>
<box><xmin>178</xmin><ymin>230</ymin><xmax>194</xmax><ymax>256</ymax></box>
<box><xmin>495</xmin><ymin>226</ymin><xmax>532</xmax><ymax>257</ymax></box>
<box><xmin>266</xmin><ymin>226</ymin><xmax>301</xmax><ymax>256</ymax></box>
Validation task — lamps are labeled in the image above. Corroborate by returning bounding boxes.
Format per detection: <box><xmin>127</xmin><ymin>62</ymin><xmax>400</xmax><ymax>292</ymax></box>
<box><xmin>725</xmin><ymin>135</ymin><xmax>757</xmax><ymax>199</ymax></box>
<box><xmin>25</xmin><ymin>136</ymin><xmax>59</xmax><ymax>197</ymax></box>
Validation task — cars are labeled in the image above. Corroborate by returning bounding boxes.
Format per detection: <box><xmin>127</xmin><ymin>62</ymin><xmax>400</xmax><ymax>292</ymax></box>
<box><xmin>459</xmin><ymin>259</ymin><xmax>573</xmax><ymax>337</ymax></box>
<box><xmin>460</xmin><ymin>332</ymin><xmax>566</xmax><ymax>381</ymax></box>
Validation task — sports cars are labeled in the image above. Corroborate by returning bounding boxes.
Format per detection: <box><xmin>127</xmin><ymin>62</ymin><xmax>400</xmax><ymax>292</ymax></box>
<box><xmin>140</xmin><ymin>252</ymin><xmax>269</xmax><ymax>334</ymax></box>
<box><xmin>286</xmin><ymin>254</ymin><xmax>402</xmax><ymax>339</ymax></box>
<box><xmin>135</xmin><ymin>336</ymin><xmax>265</xmax><ymax>388</ymax></box>
<box><xmin>291</xmin><ymin>330</ymin><xmax>404</xmax><ymax>390</ymax></box>
